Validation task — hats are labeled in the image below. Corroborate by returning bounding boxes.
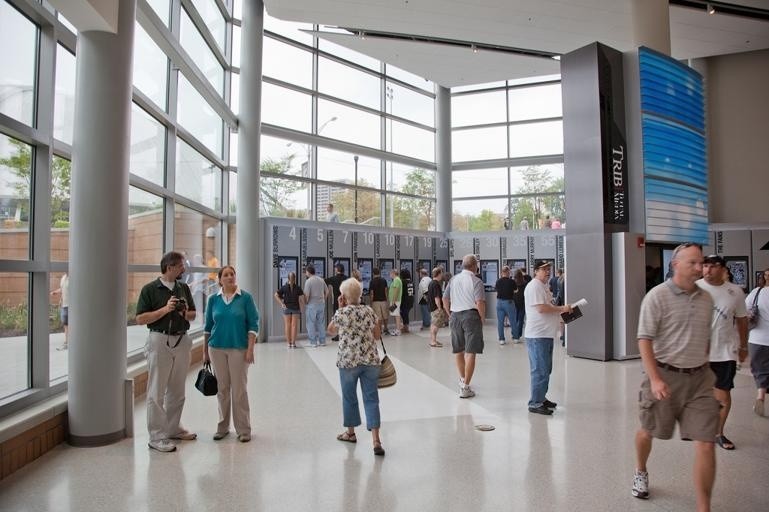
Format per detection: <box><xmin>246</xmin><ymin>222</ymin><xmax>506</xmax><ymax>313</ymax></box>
<box><xmin>536</xmin><ymin>260</ymin><xmax>553</xmax><ymax>268</ymax></box>
<box><xmin>701</xmin><ymin>255</ymin><xmax>723</xmax><ymax>263</ymax></box>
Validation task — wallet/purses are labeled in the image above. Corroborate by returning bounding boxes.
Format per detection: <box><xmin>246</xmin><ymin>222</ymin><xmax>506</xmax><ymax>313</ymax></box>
<box><xmin>389</xmin><ymin>304</ymin><xmax>397</xmax><ymax>312</ymax></box>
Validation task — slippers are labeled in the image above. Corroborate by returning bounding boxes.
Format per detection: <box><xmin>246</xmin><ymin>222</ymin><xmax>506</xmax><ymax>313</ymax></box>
<box><xmin>716</xmin><ymin>436</ymin><xmax>735</xmax><ymax>450</ymax></box>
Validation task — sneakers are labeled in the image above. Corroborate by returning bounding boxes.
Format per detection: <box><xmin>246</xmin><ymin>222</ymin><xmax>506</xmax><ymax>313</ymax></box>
<box><xmin>514</xmin><ymin>339</ymin><xmax>521</xmax><ymax>344</ymax></box>
<box><xmin>288</xmin><ymin>335</ymin><xmax>339</xmax><ymax>349</ymax></box>
<box><xmin>381</xmin><ymin>327</ymin><xmax>409</xmax><ymax>336</ymax></box>
<box><xmin>213</xmin><ymin>431</ymin><xmax>229</xmax><ymax>440</ymax></box>
<box><xmin>429</xmin><ymin>341</ymin><xmax>443</xmax><ymax>347</ymax></box>
<box><xmin>499</xmin><ymin>340</ymin><xmax>506</xmax><ymax>345</ymax></box>
<box><xmin>239</xmin><ymin>434</ymin><xmax>250</xmax><ymax>442</ymax></box>
<box><xmin>752</xmin><ymin>399</ymin><xmax>764</xmax><ymax>416</ymax></box>
<box><xmin>167</xmin><ymin>431</ymin><xmax>197</xmax><ymax>440</ymax></box>
<box><xmin>460</xmin><ymin>378</ymin><xmax>474</xmax><ymax>397</ymax></box>
<box><xmin>529</xmin><ymin>400</ymin><xmax>557</xmax><ymax>414</ymax></box>
<box><xmin>632</xmin><ymin>471</ymin><xmax>650</xmax><ymax>499</ymax></box>
<box><xmin>148</xmin><ymin>439</ymin><xmax>176</xmax><ymax>452</ymax></box>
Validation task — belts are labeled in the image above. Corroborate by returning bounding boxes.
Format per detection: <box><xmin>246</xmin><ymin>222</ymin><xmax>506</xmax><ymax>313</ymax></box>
<box><xmin>150</xmin><ymin>329</ymin><xmax>185</xmax><ymax>335</ymax></box>
<box><xmin>656</xmin><ymin>360</ymin><xmax>704</xmax><ymax>373</ymax></box>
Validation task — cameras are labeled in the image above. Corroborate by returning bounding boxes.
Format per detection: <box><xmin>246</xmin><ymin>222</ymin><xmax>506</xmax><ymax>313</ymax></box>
<box><xmin>173</xmin><ymin>294</ymin><xmax>185</xmax><ymax>311</ymax></box>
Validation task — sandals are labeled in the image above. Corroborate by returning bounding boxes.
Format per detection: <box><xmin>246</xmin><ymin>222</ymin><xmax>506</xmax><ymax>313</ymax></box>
<box><xmin>337</xmin><ymin>432</ymin><xmax>357</xmax><ymax>443</ymax></box>
<box><xmin>373</xmin><ymin>440</ymin><xmax>384</xmax><ymax>455</ymax></box>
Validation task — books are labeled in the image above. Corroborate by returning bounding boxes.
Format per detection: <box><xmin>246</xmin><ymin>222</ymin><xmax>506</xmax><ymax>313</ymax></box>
<box><xmin>561</xmin><ymin>305</ymin><xmax>582</xmax><ymax>324</ymax></box>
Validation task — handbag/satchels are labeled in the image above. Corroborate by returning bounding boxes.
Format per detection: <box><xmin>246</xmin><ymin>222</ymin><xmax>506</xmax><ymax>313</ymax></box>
<box><xmin>378</xmin><ymin>356</ymin><xmax>396</xmax><ymax>387</ymax></box>
<box><xmin>195</xmin><ymin>360</ymin><xmax>218</xmax><ymax>396</ymax></box>
<box><xmin>747</xmin><ymin>306</ymin><xmax>758</xmax><ymax>330</ymax></box>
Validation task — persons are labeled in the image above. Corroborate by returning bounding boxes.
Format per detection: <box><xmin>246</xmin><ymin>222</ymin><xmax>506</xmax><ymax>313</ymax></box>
<box><xmin>49</xmin><ymin>272</ymin><xmax>70</xmax><ymax>350</ymax></box>
<box><xmin>631</xmin><ymin>243</ymin><xmax>720</xmax><ymax>512</ymax></box>
<box><xmin>303</xmin><ymin>266</ymin><xmax>328</xmax><ymax>346</ymax></box>
<box><xmin>494</xmin><ymin>266</ymin><xmax>533</xmax><ymax>345</ymax></box>
<box><xmin>328</xmin><ymin>277</ymin><xmax>386</xmax><ymax>456</ymax></box>
<box><xmin>520</xmin><ymin>217</ymin><xmax>528</xmax><ymax>230</ymax></box>
<box><xmin>537</xmin><ymin>215</ymin><xmax>561</xmax><ymax>230</ymax></box>
<box><xmin>326</xmin><ymin>205</ymin><xmax>339</xmax><ymax>222</ymax></box>
<box><xmin>418</xmin><ymin>265</ymin><xmax>451</xmax><ymax>347</ymax></box>
<box><xmin>523</xmin><ymin>261</ymin><xmax>574</xmax><ymax>415</ymax></box>
<box><xmin>274</xmin><ymin>271</ymin><xmax>305</xmax><ymax>348</ymax></box>
<box><xmin>549</xmin><ymin>268</ymin><xmax>564</xmax><ymax>339</ymax></box>
<box><xmin>352</xmin><ymin>268</ymin><xmax>414</xmax><ymax>336</ymax></box>
<box><xmin>203</xmin><ymin>266</ymin><xmax>259</xmax><ymax>442</ymax></box>
<box><xmin>694</xmin><ymin>255</ymin><xmax>769</xmax><ymax>450</ymax></box>
<box><xmin>135</xmin><ymin>252</ymin><xmax>197</xmax><ymax>452</ymax></box>
<box><xmin>325</xmin><ymin>264</ymin><xmax>346</xmax><ymax>341</ymax></box>
<box><xmin>504</xmin><ymin>217</ymin><xmax>513</xmax><ymax>230</ymax></box>
<box><xmin>442</xmin><ymin>254</ymin><xmax>485</xmax><ymax>398</ymax></box>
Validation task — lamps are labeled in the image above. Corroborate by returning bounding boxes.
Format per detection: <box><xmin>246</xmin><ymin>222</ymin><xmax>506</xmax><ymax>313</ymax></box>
<box><xmin>471</xmin><ymin>44</ymin><xmax>480</xmax><ymax>55</ymax></box>
<box><xmin>356</xmin><ymin>31</ymin><xmax>367</xmax><ymax>41</ymax></box>
<box><xmin>706</xmin><ymin>2</ymin><xmax>716</xmax><ymax>16</ymax></box>
